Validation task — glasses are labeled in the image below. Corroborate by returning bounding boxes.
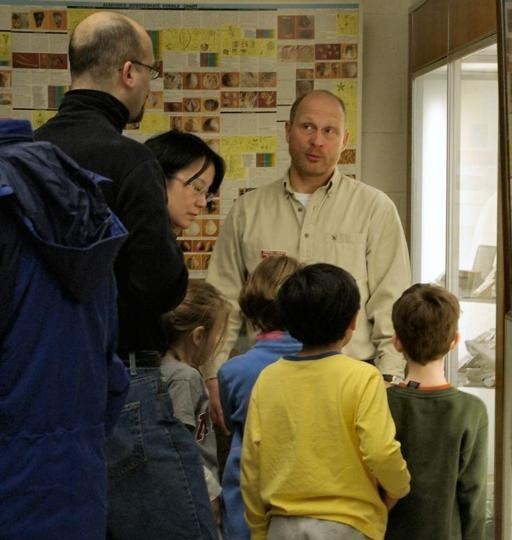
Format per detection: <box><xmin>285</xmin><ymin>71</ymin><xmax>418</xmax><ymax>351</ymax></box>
<box><xmin>168</xmin><ymin>174</ymin><xmax>215</xmax><ymax>204</ymax></box>
<box><xmin>119</xmin><ymin>58</ymin><xmax>163</xmax><ymax>80</ymax></box>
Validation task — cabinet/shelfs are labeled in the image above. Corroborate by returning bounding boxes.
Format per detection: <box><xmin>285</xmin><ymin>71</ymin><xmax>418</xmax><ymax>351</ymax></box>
<box><xmin>457</xmin><ymin>296</ymin><xmax>497</xmax><ymax>390</ymax></box>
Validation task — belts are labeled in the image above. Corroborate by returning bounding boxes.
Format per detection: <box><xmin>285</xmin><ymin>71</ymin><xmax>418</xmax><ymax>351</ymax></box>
<box><xmin>121</xmin><ymin>352</ymin><xmax>163</xmax><ymax>368</ymax></box>
